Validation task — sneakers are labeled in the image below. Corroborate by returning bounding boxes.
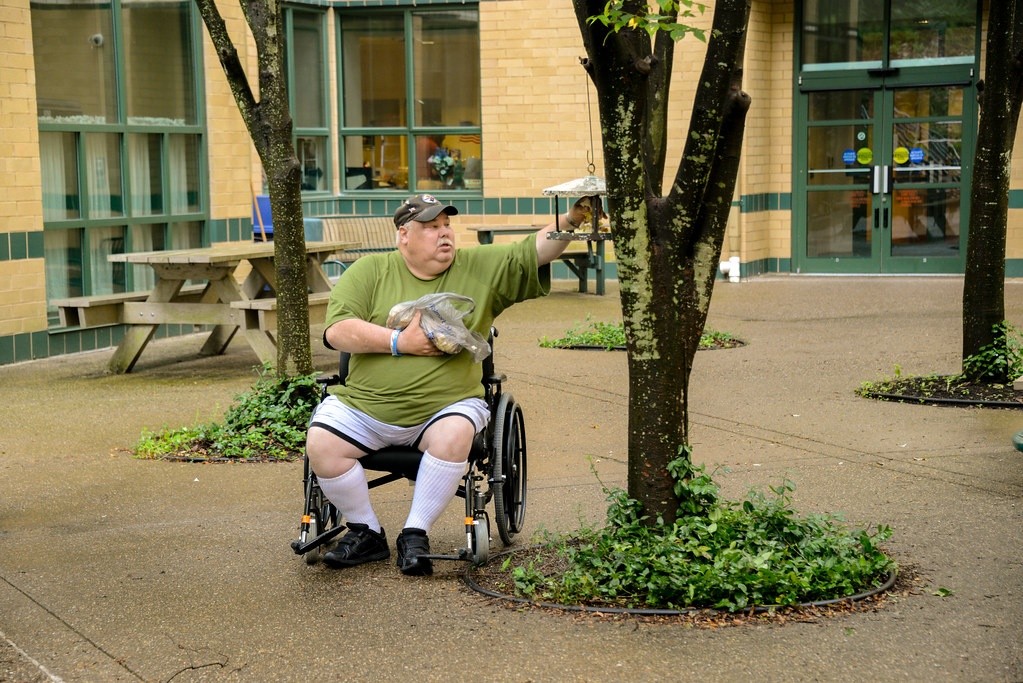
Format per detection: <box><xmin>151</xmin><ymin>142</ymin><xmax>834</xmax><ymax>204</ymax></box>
<box><xmin>322</xmin><ymin>522</ymin><xmax>390</xmax><ymax>569</ymax></box>
<box><xmin>396</xmin><ymin>528</ymin><xmax>433</xmax><ymax>576</ymax></box>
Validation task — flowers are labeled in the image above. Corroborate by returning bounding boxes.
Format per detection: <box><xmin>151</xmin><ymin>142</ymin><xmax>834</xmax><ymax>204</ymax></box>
<box><xmin>428</xmin><ymin>148</ymin><xmax>455</xmax><ymax>184</ymax></box>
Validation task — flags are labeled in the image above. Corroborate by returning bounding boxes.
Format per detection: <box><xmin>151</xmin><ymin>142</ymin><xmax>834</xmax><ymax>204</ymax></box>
<box><xmin>459</xmin><ymin>121</ymin><xmax>480</xmax><ymax>143</ymax></box>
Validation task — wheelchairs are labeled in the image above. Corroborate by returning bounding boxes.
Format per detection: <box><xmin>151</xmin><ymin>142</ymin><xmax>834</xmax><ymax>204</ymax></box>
<box><xmin>290</xmin><ymin>323</ymin><xmax>530</xmax><ymax>566</ymax></box>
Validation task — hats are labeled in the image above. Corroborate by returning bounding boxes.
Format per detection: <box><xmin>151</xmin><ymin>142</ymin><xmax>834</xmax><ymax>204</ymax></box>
<box><xmin>394</xmin><ymin>194</ymin><xmax>458</xmax><ymax>229</ymax></box>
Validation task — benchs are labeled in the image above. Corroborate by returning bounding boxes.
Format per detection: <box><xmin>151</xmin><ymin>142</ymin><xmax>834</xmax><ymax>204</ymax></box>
<box><xmin>50</xmin><ymin>283</ymin><xmax>207</xmax><ymax>329</ymax></box>
<box><xmin>230</xmin><ymin>291</ymin><xmax>332</xmax><ymax>331</ymax></box>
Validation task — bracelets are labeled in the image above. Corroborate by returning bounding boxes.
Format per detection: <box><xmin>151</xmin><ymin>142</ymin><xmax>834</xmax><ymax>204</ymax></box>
<box><xmin>390</xmin><ymin>330</ymin><xmax>402</xmax><ymax>357</ymax></box>
<box><xmin>566</xmin><ymin>212</ymin><xmax>579</xmax><ymax>228</ymax></box>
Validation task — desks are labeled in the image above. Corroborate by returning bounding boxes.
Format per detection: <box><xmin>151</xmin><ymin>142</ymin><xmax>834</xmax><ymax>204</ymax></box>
<box><xmin>465</xmin><ymin>226</ymin><xmax>612</xmax><ymax>295</ymax></box>
<box><xmin>106</xmin><ymin>241</ymin><xmax>362</xmax><ymax>380</ymax></box>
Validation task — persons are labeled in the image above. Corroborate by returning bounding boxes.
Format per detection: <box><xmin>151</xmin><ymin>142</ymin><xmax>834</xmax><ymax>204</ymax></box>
<box><xmin>416</xmin><ymin>124</ymin><xmax>446</xmax><ymax>181</ymax></box>
<box><xmin>306</xmin><ymin>195</ymin><xmax>609</xmax><ymax>575</ymax></box>
